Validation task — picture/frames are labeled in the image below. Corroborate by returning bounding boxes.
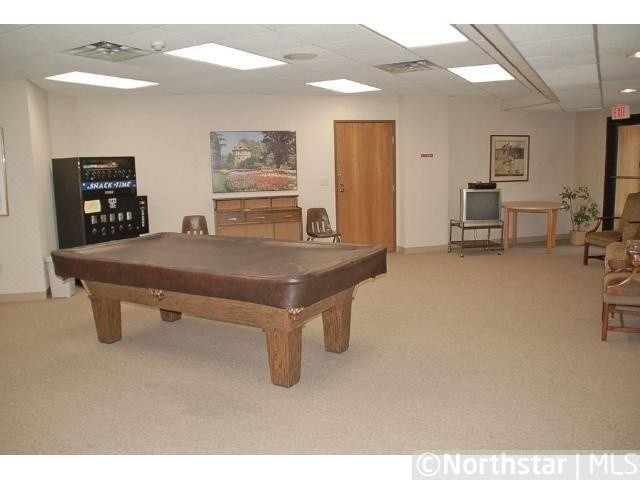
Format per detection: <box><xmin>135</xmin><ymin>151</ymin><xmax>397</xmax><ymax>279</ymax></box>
<box><xmin>489</xmin><ymin>134</ymin><xmax>531</xmax><ymax>183</ymax></box>
<box><xmin>0</xmin><ymin>126</ymin><xmax>11</xmax><ymax>219</ymax></box>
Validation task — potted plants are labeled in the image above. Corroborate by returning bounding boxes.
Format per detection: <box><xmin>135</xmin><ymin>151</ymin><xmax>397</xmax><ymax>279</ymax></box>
<box><xmin>559</xmin><ymin>183</ymin><xmax>599</xmax><ymax>246</ymax></box>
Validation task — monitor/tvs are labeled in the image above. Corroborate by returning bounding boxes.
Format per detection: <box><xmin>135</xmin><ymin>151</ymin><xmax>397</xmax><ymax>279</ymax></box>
<box><xmin>461</xmin><ymin>189</ymin><xmax>502</xmax><ymax>225</ymax></box>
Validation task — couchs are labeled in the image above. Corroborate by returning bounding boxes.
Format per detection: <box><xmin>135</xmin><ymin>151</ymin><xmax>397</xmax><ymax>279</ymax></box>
<box><xmin>601</xmin><ymin>271</ymin><xmax>640</xmax><ymax>342</ymax></box>
<box><xmin>604</xmin><ymin>240</ymin><xmax>640</xmax><ymax>271</ymax></box>
<box><xmin>583</xmin><ymin>191</ymin><xmax>639</xmax><ymax>265</ymax></box>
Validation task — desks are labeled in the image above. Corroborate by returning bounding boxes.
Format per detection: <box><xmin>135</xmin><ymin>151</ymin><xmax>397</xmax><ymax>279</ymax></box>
<box><xmin>502</xmin><ymin>200</ymin><xmax>567</xmax><ymax>254</ymax></box>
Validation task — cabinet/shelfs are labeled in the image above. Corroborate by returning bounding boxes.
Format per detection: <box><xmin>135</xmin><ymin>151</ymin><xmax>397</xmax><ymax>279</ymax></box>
<box><xmin>447</xmin><ymin>218</ymin><xmax>502</xmax><ymax>257</ymax></box>
<box><xmin>213</xmin><ymin>194</ymin><xmax>303</xmax><ymax>244</ymax></box>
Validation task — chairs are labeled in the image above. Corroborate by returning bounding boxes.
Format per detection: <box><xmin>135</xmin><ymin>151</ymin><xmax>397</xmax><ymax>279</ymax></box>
<box><xmin>304</xmin><ymin>207</ymin><xmax>342</xmax><ymax>244</ymax></box>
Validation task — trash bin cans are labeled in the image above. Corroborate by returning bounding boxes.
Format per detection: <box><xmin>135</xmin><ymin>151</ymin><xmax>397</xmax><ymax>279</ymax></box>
<box><xmin>44</xmin><ymin>258</ymin><xmax>75</xmax><ymax>298</ymax></box>
